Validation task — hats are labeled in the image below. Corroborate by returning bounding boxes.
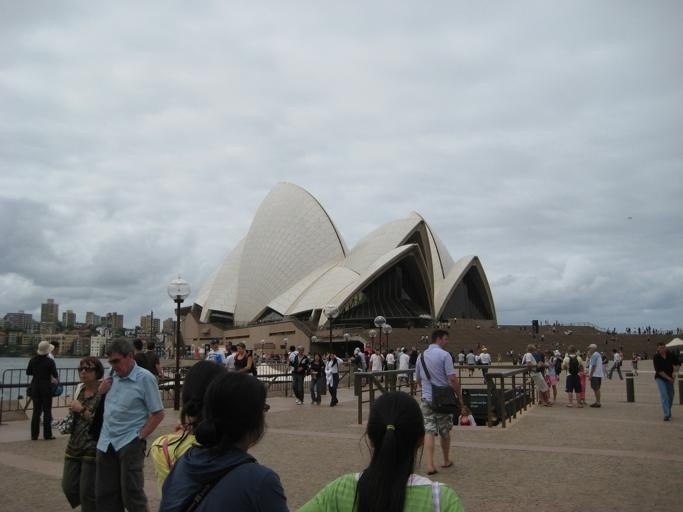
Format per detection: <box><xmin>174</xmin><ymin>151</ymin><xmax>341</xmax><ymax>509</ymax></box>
<box><xmin>36</xmin><ymin>340</ymin><xmax>55</xmax><ymax>356</ymax></box>
<box><xmin>210</xmin><ymin>339</ymin><xmax>219</xmax><ymax>344</ymax></box>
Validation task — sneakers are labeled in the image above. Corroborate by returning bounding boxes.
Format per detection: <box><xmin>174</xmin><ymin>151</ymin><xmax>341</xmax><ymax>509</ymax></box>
<box><xmin>537</xmin><ymin>398</ymin><xmax>601</xmax><ymax>408</ymax></box>
<box><xmin>295</xmin><ymin>397</ymin><xmax>338</xmax><ymax>407</ymax></box>
<box><xmin>664</xmin><ymin>416</ymin><xmax>669</xmax><ymax>421</ymax></box>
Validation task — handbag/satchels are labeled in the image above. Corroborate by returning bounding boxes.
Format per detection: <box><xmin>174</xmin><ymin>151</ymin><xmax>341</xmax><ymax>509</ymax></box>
<box><xmin>55</xmin><ymin>383</ymin><xmax>87</xmax><ymax>435</ymax></box>
<box><xmin>431</xmin><ymin>384</ymin><xmax>457</xmax><ymax>414</ymax></box>
<box><xmin>88</xmin><ymin>367</ymin><xmax>114</xmax><ymax>435</ymax></box>
<box><xmin>50</xmin><ymin>378</ymin><xmax>63</xmax><ymax>397</ymax></box>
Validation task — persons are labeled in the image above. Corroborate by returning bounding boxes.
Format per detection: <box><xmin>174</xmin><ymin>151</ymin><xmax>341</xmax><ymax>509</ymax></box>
<box><xmin>25</xmin><ymin>327</ymin><xmax>464</xmax><ymax>511</ymax></box>
<box><xmin>513</xmin><ymin>342</ymin><xmax>640</xmax><ymax>409</ymax></box>
<box><xmin>451</xmin><ymin>348</ymin><xmax>491</xmax><ymax>378</ymax></box>
<box><xmin>653</xmin><ymin>342</ymin><xmax>682</xmax><ymax>421</ymax></box>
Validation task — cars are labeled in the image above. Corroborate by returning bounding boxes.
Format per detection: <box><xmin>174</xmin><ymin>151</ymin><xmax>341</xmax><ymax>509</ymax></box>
<box><xmin>258</xmin><ymin>353</ymin><xmax>281</xmax><ymax>363</ymax></box>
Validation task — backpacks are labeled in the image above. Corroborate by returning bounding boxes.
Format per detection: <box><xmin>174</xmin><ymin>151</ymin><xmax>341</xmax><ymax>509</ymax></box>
<box><xmin>567</xmin><ymin>355</ymin><xmax>580</xmax><ymax>377</ymax></box>
<box><xmin>551</xmin><ymin>357</ymin><xmax>561</xmax><ymax>376</ymax></box>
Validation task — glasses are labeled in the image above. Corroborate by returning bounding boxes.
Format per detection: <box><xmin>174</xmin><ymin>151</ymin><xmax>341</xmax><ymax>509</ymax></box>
<box><xmin>262</xmin><ymin>403</ymin><xmax>270</xmax><ymax>412</ymax></box>
<box><xmin>78</xmin><ymin>366</ymin><xmax>97</xmax><ymax>373</ymax></box>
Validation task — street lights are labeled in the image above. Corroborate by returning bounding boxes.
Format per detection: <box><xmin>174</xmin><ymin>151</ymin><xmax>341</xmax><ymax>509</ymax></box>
<box><xmin>260</xmin><ymin>339</ymin><xmax>264</xmax><ymax>364</ymax></box>
<box><xmin>283</xmin><ymin>337</ymin><xmax>288</xmax><ymax>365</ymax></box>
<box><xmin>310</xmin><ymin>315</ymin><xmax>394</xmax><ymax>359</ymax></box>
<box><xmin>164</xmin><ymin>270</ymin><xmax>193</xmax><ymax>411</ymax></box>
<box><xmin>321</xmin><ymin>303</ymin><xmax>339</xmax><ymax>355</ymax></box>
